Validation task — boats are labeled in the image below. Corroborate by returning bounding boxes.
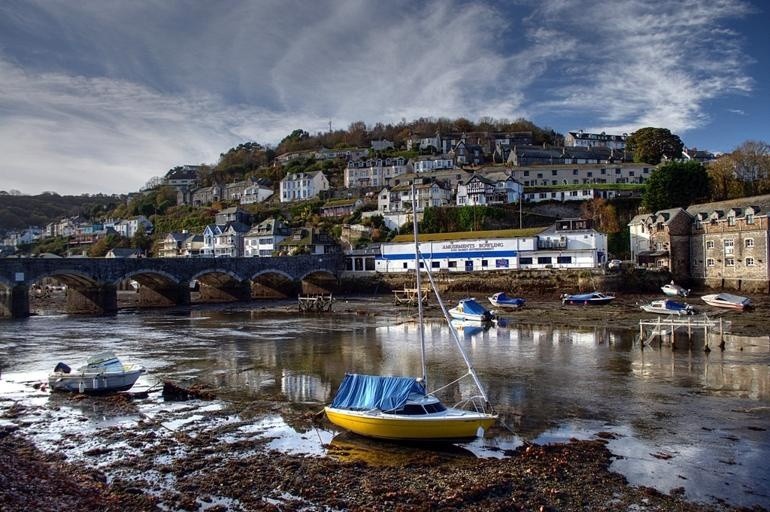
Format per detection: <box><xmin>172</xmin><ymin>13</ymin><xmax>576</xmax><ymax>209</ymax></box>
<box><xmin>641</xmin><ymin>280</ymin><xmax>753</xmax><ymax>317</ymax></box>
<box><xmin>448</xmin><ymin>291</ymin><xmax>526</xmax><ymax>321</ymax></box>
<box><xmin>560</xmin><ymin>291</ymin><xmax>616</xmax><ymax>305</ymax></box>
<box><xmin>49</xmin><ymin>350</ymin><xmax>143</xmax><ymax>393</ymax></box>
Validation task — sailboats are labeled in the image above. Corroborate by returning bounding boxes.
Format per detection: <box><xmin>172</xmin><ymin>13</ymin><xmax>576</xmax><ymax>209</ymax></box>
<box><xmin>324</xmin><ymin>184</ymin><xmax>498</xmax><ymax>441</ymax></box>
